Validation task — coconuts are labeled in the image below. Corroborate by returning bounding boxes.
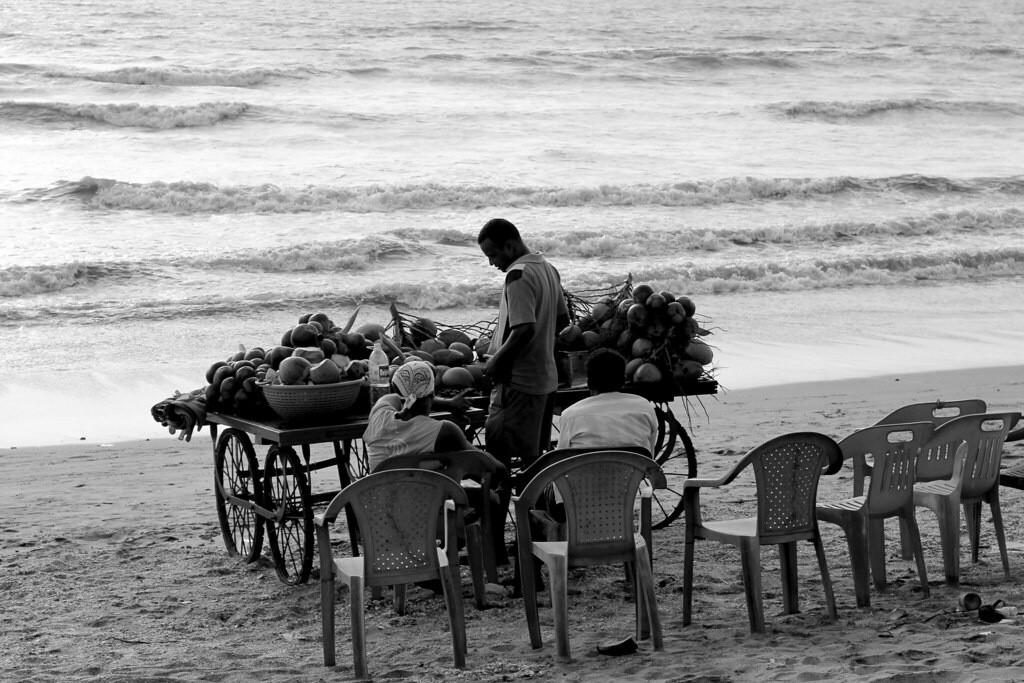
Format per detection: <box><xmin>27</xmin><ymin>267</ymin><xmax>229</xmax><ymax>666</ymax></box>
<box><xmin>205</xmin><ymin>285</ymin><xmax>713</xmax><ymax>407</ymax></box>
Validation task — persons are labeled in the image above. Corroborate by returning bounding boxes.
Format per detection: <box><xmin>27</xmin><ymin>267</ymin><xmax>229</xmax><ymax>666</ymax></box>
<box><xmin>507</xmin><ymin>348</ymin><xmax>659</xmax><ymax>598</ymax></box>
<box><xmin>477</xmin><ymin>218</ymin><xmax>570</xmax><ymax>592</ymax></box>
<box><xmin>363</xmin><ymin>361</ymin><xmax>509</xmax><ymax>552</ymax></box>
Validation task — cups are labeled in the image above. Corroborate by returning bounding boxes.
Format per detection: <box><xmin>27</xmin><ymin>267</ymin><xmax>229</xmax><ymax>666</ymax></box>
<box><xmin>958</xmin><ymin>592</ymin><xmax>981</xmax><ymax>611</ymax></box>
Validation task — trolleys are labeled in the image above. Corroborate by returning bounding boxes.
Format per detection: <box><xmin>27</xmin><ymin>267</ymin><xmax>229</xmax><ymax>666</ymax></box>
<box><xmin>204</xmin><ymin>373</ymin><xmax>719</xmax><ymax>586</ymax></box>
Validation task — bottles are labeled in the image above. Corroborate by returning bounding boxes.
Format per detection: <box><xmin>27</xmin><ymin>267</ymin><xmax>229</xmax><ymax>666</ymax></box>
<box><xmin>366</xmin><ymin>343</ymin><xmax>391</xmax><ymax>409</ymax></box>
<box><xmin>995</xmin><ymin>607</ymin><xmax>1017</xmax><ymax>616</ymax></box>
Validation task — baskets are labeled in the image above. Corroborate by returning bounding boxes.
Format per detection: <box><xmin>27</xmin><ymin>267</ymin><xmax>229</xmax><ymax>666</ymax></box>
<box><xmin>255</xmin><ymin>378</ymin><xmax>365</xmax><ymax>421</ymax></box>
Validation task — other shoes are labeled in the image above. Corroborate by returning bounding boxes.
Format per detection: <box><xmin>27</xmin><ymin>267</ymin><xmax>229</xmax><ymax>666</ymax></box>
<box><xmin>501</xmin><ymin>577</ymin><xmax>544</xmax><ymax>598</ymax></box>
<box><xmin>416</xmin><ymin>579</ymin><xmax>444</xmax><ymax>594</ymax></box>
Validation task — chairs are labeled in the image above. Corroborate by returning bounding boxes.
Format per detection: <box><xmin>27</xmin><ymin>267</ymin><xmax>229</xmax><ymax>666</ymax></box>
<box><xmin>681</xmin><ymin>399</ymin><xmax>1024</xmax><ymax>633</ymax></box>
<box><xmin>370</xmin><ymin>450</ymin><xmax>496</xmax><ymax>615</ymax></box>
<box><xmin>517</xmin><ymin>446</ymin><xmax>653</xmax><ymax>596</ymax></box>
<box><xmin>512</xmin><ymin>450</ymin><xmax>668</xmax><ymax>664</ymax></box>
<box><xmin>313</xmin><ymin>468</ymin><xmax>470</xmax><ymax>678</ymax></box>
